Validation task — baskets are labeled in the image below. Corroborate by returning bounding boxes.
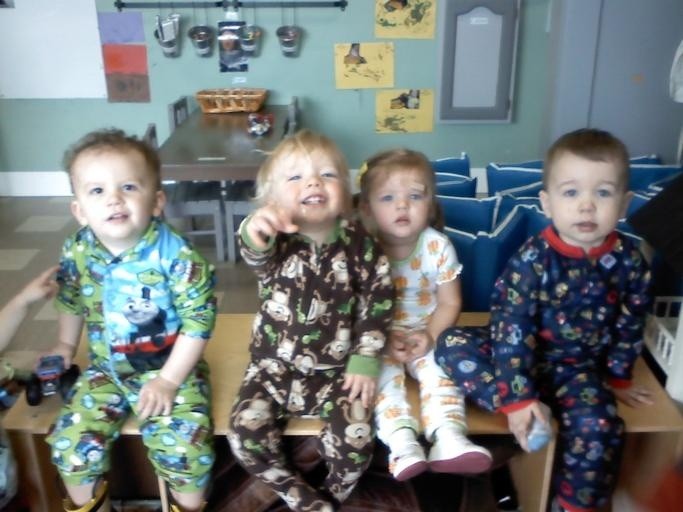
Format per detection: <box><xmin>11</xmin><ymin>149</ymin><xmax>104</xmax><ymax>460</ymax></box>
<box><xmin>194</xmin><ymin>89</ymin><xmax>267</xmax><ymax>114</ymax></box>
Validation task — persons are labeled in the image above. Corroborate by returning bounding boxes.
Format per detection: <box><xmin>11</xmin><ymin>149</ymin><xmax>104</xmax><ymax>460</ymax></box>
<box><xmin>353</xmin><ymin>147</ymin><xmax>492</xmax><ymax>483</ymax></box>
<box><xmin>0</xmin><ymin>260</ymin><xmax>65</xmax><ymax>512</ymax></box>
<box><xmin>224</xmin><ymin>127</ymin><xmax>396</xmax><ymax>512</ymax></box>
<box><xmin>434</xmin><ymin>129</ymin><xmax>656</xmax><ymax>512</ymax></box>
<box><xmin>42</xmin><ymin>123</ymin><xmax>221</xmax><ymax>512</ymax></box>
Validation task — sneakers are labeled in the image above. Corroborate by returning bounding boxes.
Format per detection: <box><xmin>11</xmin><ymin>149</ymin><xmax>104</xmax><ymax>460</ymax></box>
<box><xmin>388</xmin><ymin>437</ymin><xmax>493</xmax><ymax>481</ymax></box>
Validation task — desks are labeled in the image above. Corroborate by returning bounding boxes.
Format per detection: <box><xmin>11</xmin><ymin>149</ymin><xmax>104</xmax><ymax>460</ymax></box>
<box><xmin>0</xmin><ymin>315</ymin><xmax>683</xmax><ymax>512</ymax></box>
<box><xmin>158</xmin><ymin>105</ymin><xmax>289</xmax><ymax>230</ymax></box>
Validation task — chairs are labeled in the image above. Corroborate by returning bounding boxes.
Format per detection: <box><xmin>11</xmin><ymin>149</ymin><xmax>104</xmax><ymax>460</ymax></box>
<box><xmin>224</xmin><ymin>122</ymin><xmax>297</xmax><ymax>263</ymax></box>
<box><xmin>144</xmin><ymin>123</ymin><xmax>224</xmax><ymax>263</ymax></box>
<box><xmin>168</xmin><ymin>96</ymin><xmax>188</xmax><ymax>130</ymax></box>
<box><xmin>288</xmin><ymin>95</ymin><xmax>300</xmax><ymax>121</ymax></box>
